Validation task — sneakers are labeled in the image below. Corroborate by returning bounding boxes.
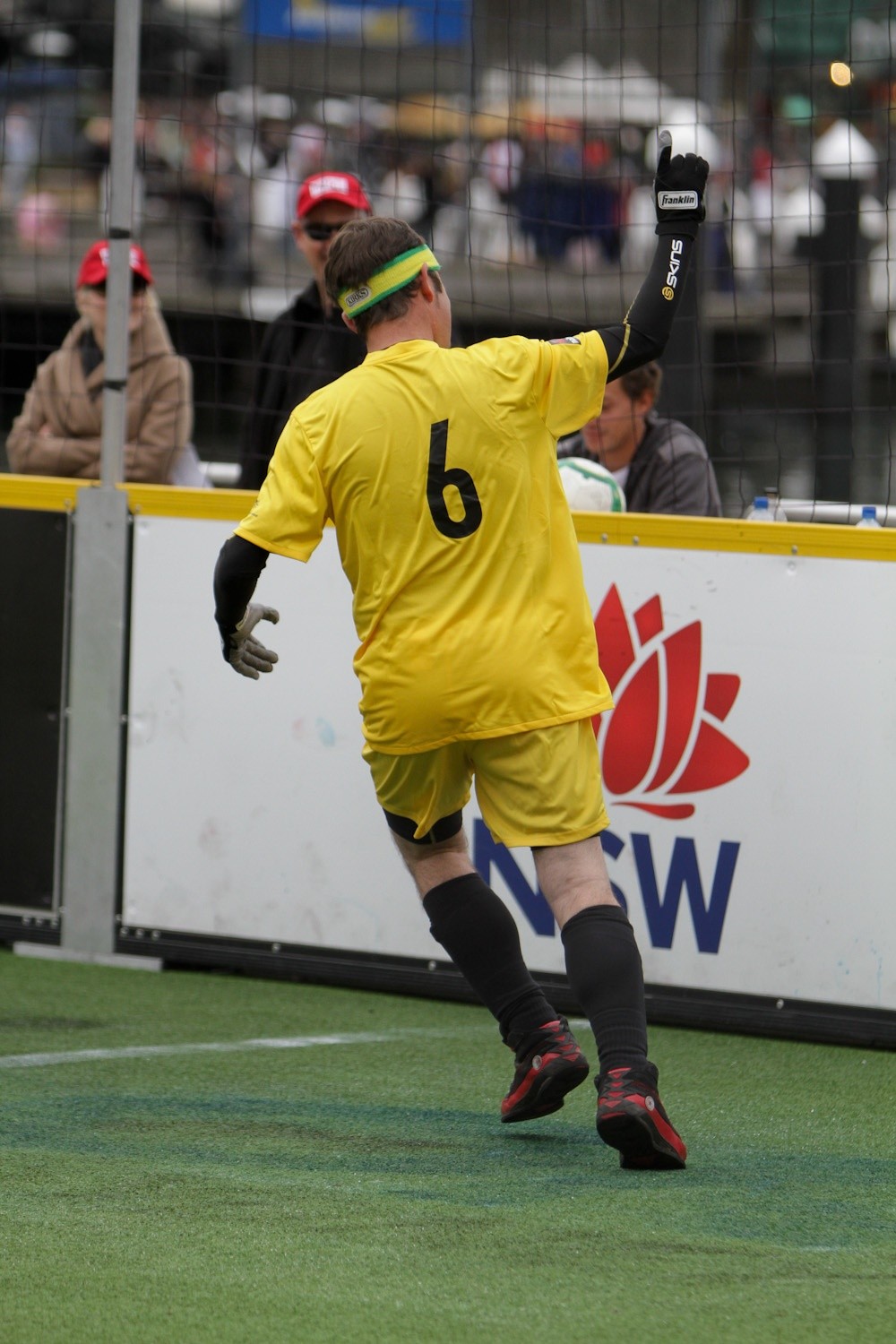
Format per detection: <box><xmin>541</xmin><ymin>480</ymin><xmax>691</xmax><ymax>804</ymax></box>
<box><xmin>500</xmin><ymin>1017</ymin><xmax>588</xmax><ymax>1123</ymax></box>
<box><xmin>592</xmin><ymin>1061</ymin><xmax>687</xmax><ymax>1170</ymax></box>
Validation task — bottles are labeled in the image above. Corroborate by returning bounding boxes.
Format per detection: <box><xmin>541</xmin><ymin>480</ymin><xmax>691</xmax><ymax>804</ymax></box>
<box><xmin>763</xmin><ymin>487</ymin><xmax>788</xmax><ymax>522</ymax></box>
<box><xmin>747</xmin><ymin>496</ymin><xmax>774</xmax><ymax>521</ymax></box>
<box><xmin>855</xmin><ymin>506</ymin><xmax>881</xmax><ymax>527</ymax></box>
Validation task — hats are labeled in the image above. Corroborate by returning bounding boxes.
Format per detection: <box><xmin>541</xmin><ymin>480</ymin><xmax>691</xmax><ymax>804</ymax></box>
<box><xmin>79</xmin><ymin>241</ymin><xmax>152</xmax><ymax>285</ymax></box>
<box><xmin>294</xmin><ymin>172</ymin><xmax>373</xmax><ymax>219</ymax></box>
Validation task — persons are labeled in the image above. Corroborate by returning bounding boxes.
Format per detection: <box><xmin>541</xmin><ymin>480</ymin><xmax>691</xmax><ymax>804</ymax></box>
<box><xmin>235</xmin><ymin>171</ymin><xmax>373</xmax><ymax>490</ymax></box>
<box><xmin>214</xmin><ymin>147</ymin><xmax>711</xmax><ymax>1170</ymax></box>
<box><xmin>6</xmin><ymin>240</ymin><xmax>192</xmax><ymax>485</ymax></box>
<box><xmin>0</xmin><ymin>93</ymin><xmax>896</xmax><ymax>292</ymax></box>
<box><xmin>556</xmin><ymin>359</ymin><xmax>722</xmax><ymax>516</ymax></box>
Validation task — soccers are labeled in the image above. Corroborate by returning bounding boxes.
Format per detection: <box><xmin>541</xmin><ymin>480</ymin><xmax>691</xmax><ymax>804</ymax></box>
<box><xmin>557</xmin><ymin>457</ymin><xmax>626</xmax><ymax>512</ymax></box>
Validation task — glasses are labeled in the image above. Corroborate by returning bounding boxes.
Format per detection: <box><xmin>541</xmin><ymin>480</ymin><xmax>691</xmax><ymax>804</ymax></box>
<box><xmin>91</xmin><ymin>276</ymin><xmax>150</xmax><ymax>296</ymax></box>
<box><xmin>299</xmin><ymin>223</ymin><xmax>352</xmax><ymax>241</ymax></box>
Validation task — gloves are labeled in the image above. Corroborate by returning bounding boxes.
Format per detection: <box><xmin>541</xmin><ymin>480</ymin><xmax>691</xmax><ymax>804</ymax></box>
<box><xmin>216</xmin><ymin>603</ymin><xmax>280</xmax><ymax>679</ymax></box>
<box><xmin>651</xmin><ymin>130</ymin><xmax>710</xmax><ymax>238</ymax></box>
